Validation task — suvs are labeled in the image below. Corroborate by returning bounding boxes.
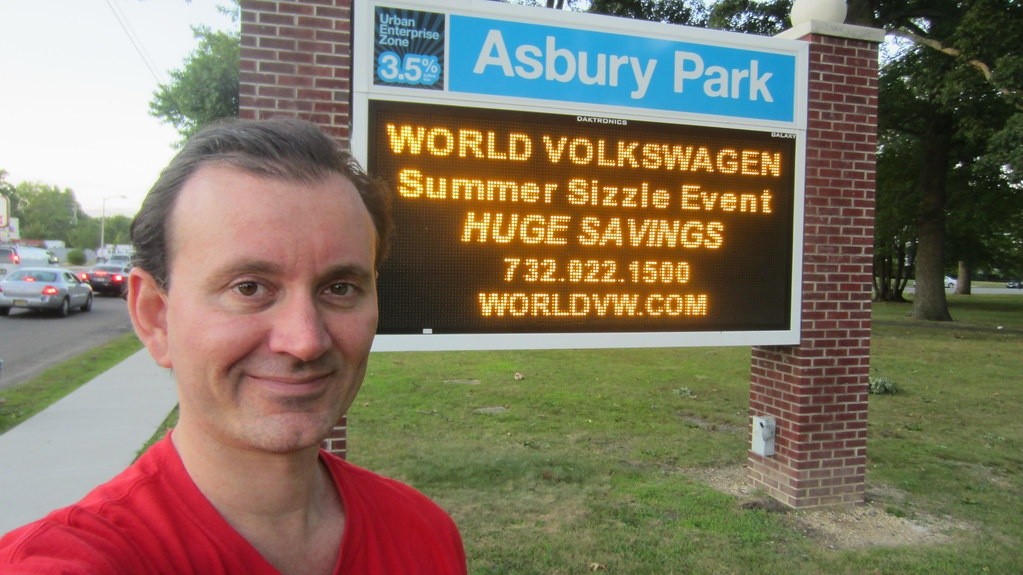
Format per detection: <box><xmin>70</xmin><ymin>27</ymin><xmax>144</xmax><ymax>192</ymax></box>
<box><xmin>1</xmin><ymin>242</ymin><xmax>62</xmax><ymax>278</ymax></box>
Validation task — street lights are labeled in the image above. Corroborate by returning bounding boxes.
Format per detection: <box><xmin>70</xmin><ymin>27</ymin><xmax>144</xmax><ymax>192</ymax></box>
<box><xmin>98</xmin><ymin>195</ymin><xmax>132</xmax><ymax>263</ymax></box>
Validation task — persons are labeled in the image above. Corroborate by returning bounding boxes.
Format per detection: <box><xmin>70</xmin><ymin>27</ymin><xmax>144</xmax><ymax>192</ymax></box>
<box><xmin>1</xmin><ymin>118</ymin><xmax>467</xmax><ymax>575</ymax></box>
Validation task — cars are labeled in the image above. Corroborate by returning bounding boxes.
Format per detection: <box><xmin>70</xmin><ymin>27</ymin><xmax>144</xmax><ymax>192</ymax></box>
<box><xmin>944</xmin><ymin>275</ymin><xmax>958</xmax><ymax>288</ymax></box>
<box><xmin>0</xmin><ymin>265</ymin><xmax>95</xmax><ymax>318</ymax></box>
<box><xmin>1007</xmin><ymin>280</ymin><xmax>1023</xmax><ymax>289</ymax></box>
<box><xmin>82</xmin><ymin>242</ymin><xmax>136</xmax><ymax>299</ymax></box>
